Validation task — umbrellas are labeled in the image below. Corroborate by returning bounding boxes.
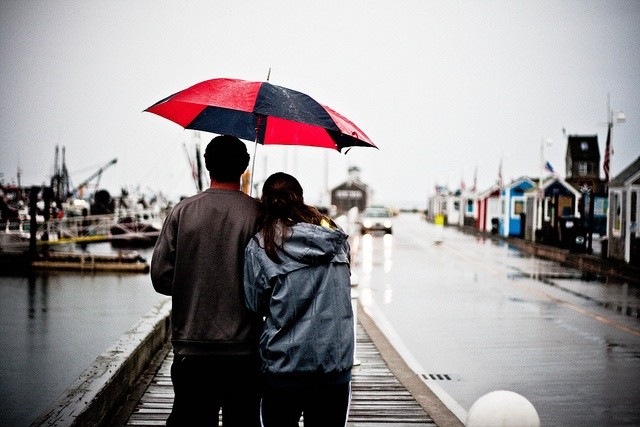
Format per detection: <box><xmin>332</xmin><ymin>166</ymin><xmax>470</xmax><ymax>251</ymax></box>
<box><xmin>141</xmin><ymin>67</ymin><xmax>382</xmax><ymax>197</ymax></box>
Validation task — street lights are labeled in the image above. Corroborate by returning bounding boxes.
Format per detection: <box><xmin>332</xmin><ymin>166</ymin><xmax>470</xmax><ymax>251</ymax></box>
<box><xmin>604</xmin><ymin>112</ymin><xmax>625</xmax><ymax>258</ymax></box>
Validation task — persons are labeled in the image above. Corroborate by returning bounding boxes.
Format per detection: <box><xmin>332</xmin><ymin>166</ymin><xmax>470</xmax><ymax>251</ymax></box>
<box><xmin>150</xmin><ymin>135</ymin><xmax>264</xmax><ymax>427</ymax></box>
<box><xmin>241</xmin><ymin>171</ymin><xmax>354</xmax><ymax>426</ymax></box>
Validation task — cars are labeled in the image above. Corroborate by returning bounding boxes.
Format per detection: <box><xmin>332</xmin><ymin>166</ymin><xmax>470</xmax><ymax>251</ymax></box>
<box><xmin>358</xmin><ymin>204</ymin><xmax>392</xmax><ymax>233</ymax></box>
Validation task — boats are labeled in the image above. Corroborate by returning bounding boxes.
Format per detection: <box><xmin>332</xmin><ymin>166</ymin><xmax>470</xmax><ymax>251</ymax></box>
<box><xmin>31</xmin><ymin>249</ymin><xmax>149</xmax><ymax>271</ymax></box>
